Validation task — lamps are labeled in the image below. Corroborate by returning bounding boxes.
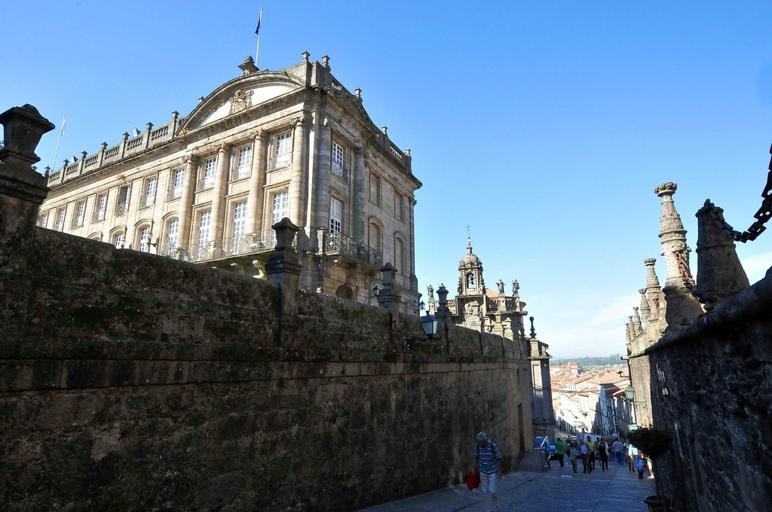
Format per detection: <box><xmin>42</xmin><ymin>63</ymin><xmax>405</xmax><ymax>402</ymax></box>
<box><xmin>420</xmin><ymin>311</ymin><xmax>439</xmax><ymax>341</ymax></box>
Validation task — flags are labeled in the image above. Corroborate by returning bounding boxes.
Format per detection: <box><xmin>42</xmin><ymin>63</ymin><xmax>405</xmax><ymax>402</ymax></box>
<box><xmin>254</xmin><ymin>11</ymin><xmax>265</xmax><ymax>39</ymax></box>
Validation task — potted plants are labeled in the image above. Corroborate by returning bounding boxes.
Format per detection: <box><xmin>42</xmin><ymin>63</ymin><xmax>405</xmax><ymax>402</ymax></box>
<box><xmin>627</xmin><ymin>426</ymin><xmax>673</xmax><ymax>511</ymax></box>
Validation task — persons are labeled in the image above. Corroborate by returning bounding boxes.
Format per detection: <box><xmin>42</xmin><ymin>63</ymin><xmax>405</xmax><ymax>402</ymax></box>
<box><xmin>555</xmin><ymin>435</ymin><xmax>646</xmax><ymax>479</ymax></box>
<box><xmin>472</xmin><ymin>431</ymin><xmax>502</xmax><ymax>511</ymax></box>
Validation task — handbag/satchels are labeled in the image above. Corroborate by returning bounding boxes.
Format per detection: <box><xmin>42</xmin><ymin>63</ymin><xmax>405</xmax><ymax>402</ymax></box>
<box><xmin>467</xmin><ymin>472</ymin><xmax>480</xmax><ymax>490</ymax></box>
